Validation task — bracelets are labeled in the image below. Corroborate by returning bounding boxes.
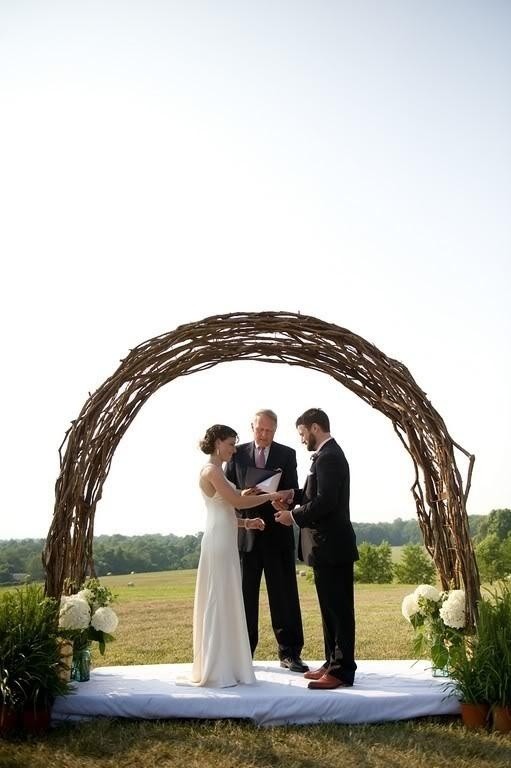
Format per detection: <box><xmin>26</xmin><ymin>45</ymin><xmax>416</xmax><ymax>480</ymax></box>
<box><xmin>245</xmin><ymin>517</ymin><xmax>251</xmax><ymax>530</ymax></box>
<box><xmin>288</xmin><ymin>488</ymin><xmax>294</xmax><ymax>504</ymax></box>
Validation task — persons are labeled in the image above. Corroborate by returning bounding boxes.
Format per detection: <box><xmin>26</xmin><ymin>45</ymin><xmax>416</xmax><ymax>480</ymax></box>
<box><xmin>176</xmin><ymin>425</ymin><xmax>284</xmax><ymax>687</ymax></box>
<box><xmin>225</xmin><ymin>409</ymin><xmax>311</xmax><ymax>673</ymax></box>
<box><xmin>272</xmin><ymin>407</ymin><xmax>360</xmax><ymax>688</ymax></box>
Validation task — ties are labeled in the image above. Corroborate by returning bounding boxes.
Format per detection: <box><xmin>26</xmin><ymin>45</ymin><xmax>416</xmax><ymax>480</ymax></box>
<box><xmin>256</xmin><ymin>447</ymin><xmax>266</xmax><ymax>466</ymax></box>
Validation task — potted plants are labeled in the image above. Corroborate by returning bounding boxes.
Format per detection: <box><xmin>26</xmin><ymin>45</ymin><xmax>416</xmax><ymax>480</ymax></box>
<box><xmin>441</xmin><ymin>584</ymin><xmax>511</xmax><ymax>731</ymax></box>
<box><xmin>0</xmin><ymin>582</ymin><xmax>75</xmax><ymax>735</ymax></box>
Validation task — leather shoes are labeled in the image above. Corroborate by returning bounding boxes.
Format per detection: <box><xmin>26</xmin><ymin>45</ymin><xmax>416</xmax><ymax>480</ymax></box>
<box><xmin>303</xmin><ymin>663</ymin><xmax>329</xmax><ymax>680</ymax></box>
<box><xmin>279</xmin><ymin>655</ymin><xmax>310</xmax><ymax>673</ymax></box>
<box><xmin>308</xmin><ymin>668</ymin><xmax>353</xmax><ymax>690</ymax></box>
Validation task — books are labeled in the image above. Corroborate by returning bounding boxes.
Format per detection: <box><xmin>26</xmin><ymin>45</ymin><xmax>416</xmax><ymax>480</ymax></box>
<box><xmin>245</xmin><ymin>464</ymin><xmax>283</xmax><ymax>494</ymax></box>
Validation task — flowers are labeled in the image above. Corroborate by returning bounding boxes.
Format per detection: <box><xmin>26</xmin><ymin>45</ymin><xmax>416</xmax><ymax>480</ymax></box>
<box><xmin>395</xmin><ymin>577</ymin><xmax>487</xmax><ymax>655</ymax></box>
<box><xmin>46</xmin><ymin>586</ymin><xmax>118</xmax><ymax>650</ymax></box>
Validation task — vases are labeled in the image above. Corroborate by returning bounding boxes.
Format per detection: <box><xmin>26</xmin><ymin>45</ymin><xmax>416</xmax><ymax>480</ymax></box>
<box><xmin>432</xmin><ymin>655</ymin><xmax>447</xmax><ymax>677</ymax></box>
<box><xmin>71</xmin><ymin>650</ymin><xmax>90</xmax><ymax>681</ymax></box>
